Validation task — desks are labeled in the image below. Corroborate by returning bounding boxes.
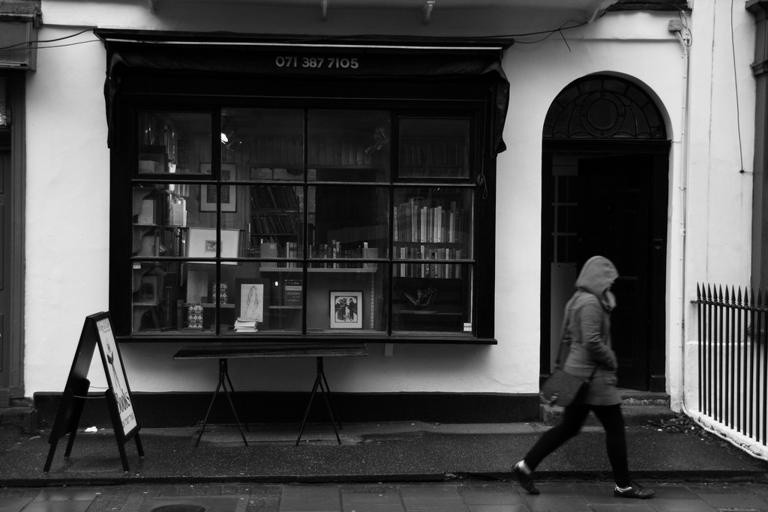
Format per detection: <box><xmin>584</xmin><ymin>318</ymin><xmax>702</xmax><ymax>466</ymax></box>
<box><xmin>170</xmin><ymin>344</ymin><xmax>371</xmax><ymax>451</ymax></box>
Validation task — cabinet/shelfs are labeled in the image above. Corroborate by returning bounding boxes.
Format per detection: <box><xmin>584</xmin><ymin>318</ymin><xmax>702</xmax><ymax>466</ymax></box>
<box><xmin>383</xmin><ymin>178</ymin><xmax>476</xmax><ymax>333</ymax></box>
<box><xmin>185</xmin><ymin>260</ymin><xmax>378</xmax><ymax>332</ymax></box>
<box><xmin>238</xmin><ymin>173</ymin><xmax>315</xmax><ymax>259</ymax></box>
<box><xmin>133</xmin><ymin>117</ymin><xmax>196</xmax><ymax>333</ymax></box>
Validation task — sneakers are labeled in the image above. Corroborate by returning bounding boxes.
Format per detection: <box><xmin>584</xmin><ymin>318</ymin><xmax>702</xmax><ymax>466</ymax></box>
<box><xmin>510</xmin><ymin>462</ymin><xmax>540</xmax><ymax>494</ymax></box>
<box><xmin>613</xmin><ymin>482</ymin><xmax>653</xmax><ymax>499</ymax></box>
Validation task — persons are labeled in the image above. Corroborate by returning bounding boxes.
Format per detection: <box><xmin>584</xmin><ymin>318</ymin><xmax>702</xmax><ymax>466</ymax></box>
<box><xmin>347</xmin><ymin>298</ymin><xmax>355</xmax><ymax>320</ymax></box>
<box><xmin>515</xmin><ymin>253</ymin><xmax>657</xmax><ymax>500</ymax></box>
<box><xmin>243</xmin><ymin>287</ymin><xmax>260</xmax><ymax>316</ymax></box>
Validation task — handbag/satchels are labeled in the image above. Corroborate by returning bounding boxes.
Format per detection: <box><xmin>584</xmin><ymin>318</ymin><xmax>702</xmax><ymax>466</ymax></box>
<box><xmin>539</xmin><ymin>371</ymin><xmax>588</xmax><ymax>406</ymax></box>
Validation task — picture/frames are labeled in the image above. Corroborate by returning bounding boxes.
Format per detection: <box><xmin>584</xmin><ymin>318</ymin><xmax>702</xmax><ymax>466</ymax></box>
<box><xmin>186</xmin><ymin>225</ymin><xmax>241</xmax><ymax>267</ymax></box>
<box><xmin>327</xmin><ymin>288</ymin><xmax>366</xmax><ymax>331</ymax></box>
<box><xmin>198</xmin><ymin>160</ymin><xmax>238</xmax><ymax>214</ymax></box>
<box><xmin>233</xmin><ymin>276</ymin><xmax>271</xmax><ymax>330</ymax></box>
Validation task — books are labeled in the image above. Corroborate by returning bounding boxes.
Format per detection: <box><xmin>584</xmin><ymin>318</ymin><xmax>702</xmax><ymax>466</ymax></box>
<box><xmin>252</xmin><ymin>186</ymin><xmax>300</xmax><ymax>249</ymax></box>
<box><xmin>233</xmin><ymin>317</ymin><xmax>259</xmax><ymax>334</ymax></box>
<box><xmin>392</xmin><ymin>196</ymin><xmax>470</xmax><ymax>279</ymax></box>
<box><xmin>278</xmin><ymin>240</ymin><xmax>362</xmax><ymax>268</ymax></box>
